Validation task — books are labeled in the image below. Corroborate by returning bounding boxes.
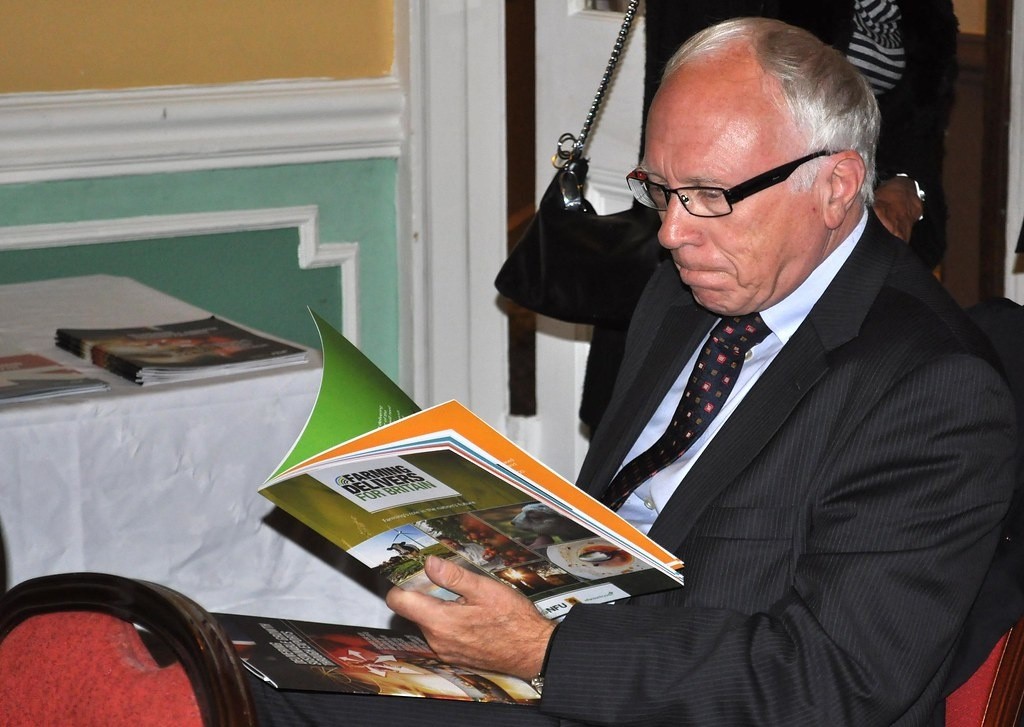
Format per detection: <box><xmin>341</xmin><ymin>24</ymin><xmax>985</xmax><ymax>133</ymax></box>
<box><xmin>257</xmin><ymin>303</ymin><xmax>687</xmax><ymax>627</ymax></box>
<box><xmin>201</xmin><ymin>605</ymin><xmax>545</xmax><ymax>706</ymax></box>
<box><xmin>1</xmin><ymin>293</ymin><xmax>310</xmax><ymax>408</ymax></box>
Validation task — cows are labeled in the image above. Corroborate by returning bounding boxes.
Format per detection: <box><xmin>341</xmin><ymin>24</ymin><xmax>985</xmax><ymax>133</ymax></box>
<box><xmin>386</xmin><ymin>541</ymin><xmax>427</xmax><ymax>566</ymax></box>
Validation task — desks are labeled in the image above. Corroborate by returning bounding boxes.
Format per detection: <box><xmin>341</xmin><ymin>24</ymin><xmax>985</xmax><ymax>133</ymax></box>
<box><xmin>1</xmin><ymin>270</ymin><xmax>397</xmax><ymax>631</ymax></box>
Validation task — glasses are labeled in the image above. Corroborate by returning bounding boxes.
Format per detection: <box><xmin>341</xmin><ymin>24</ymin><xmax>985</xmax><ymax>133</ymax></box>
<box><xmin>625</xmin><ymin>149</ymin><xmax>843</xmax><ymax>217</ymax></box>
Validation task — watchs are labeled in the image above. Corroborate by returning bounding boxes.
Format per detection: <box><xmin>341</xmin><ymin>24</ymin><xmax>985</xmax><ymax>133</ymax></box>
<box><xmin>892</xmin><ymin>168</ymin><xmax>928</xmax><ymax>221</ymax></box>
<box><xmin>531</xmin><ymin>623</ymin><xmax>560</xmax><ymax>699</ymax></box>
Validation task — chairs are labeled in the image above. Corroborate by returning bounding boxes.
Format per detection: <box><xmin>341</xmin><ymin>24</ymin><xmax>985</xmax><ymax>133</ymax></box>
<box><xmin>1</xmin><ymin>571</ymin><xmax>253</xmax><ymax>726</ymax></box>
<box><xmin>939</xmin><ymin>296</ymin><xmax>1024</xmax><ymax>726</ymax></box>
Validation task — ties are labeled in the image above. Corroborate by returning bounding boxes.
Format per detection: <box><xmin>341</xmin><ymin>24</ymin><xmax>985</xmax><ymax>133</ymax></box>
<box><xmin>600</xmin><ymin>312</ymin><xmax>772</xmax><ymax>515</ymax></box>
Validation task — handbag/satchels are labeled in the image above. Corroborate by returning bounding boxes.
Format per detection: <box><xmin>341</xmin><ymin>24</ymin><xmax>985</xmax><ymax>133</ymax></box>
<box><xmin>492</xmin><ymin>132</ymin><xmax>671</xmax><ymax>326</ymax></box>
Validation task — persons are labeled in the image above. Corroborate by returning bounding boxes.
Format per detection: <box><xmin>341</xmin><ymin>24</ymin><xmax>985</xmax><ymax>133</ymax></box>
<box><xmin>233</xmin><ymin>14</ymin><xmax>1016</xmax><ymax>727</ymax></box>
<box><xmin>671</xmin><ymin>0</ymin><xmax>966</xmax><ymax>310</ymax></box>
